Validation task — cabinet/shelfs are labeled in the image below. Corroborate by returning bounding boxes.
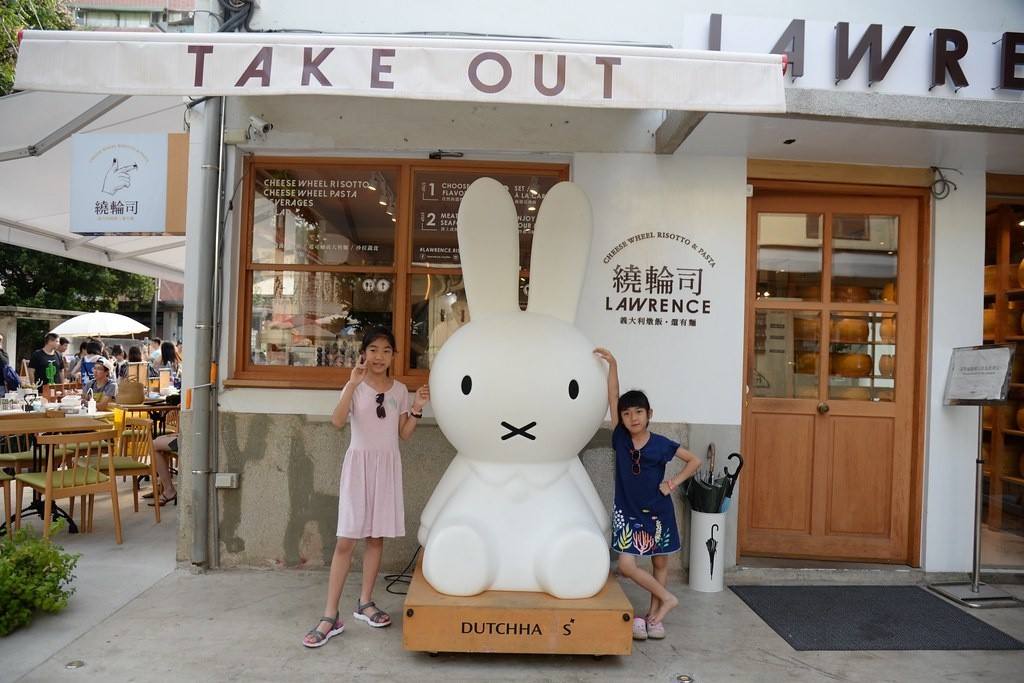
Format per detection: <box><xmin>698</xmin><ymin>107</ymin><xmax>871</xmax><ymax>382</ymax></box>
<box><xmin>980</xmin><ymin>200</ymin><xmax>1024</xmax><ymax>535</ymax></box>
<box><xmin>43</xmin><ymin>383</ymin><xmax>82</xmax><ymax>402</ymax></box>
<box><xmin>789</xmin><ymin>316</ymin><xmax>897</xmax><ymax>379</ymax></box>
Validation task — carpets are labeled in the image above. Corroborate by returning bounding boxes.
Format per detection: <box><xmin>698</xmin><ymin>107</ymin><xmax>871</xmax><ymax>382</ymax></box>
<box><xmin>727</xmin><ymin>585</ymin><xmax>1024</xmax><ymax>652</ymax></box>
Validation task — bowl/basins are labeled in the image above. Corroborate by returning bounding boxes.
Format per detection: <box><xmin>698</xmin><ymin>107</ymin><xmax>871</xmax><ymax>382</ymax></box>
<box><xmin>44</xmin><ymin>403</ymin><xmax>61</xmax><ymax>411</ymax></box>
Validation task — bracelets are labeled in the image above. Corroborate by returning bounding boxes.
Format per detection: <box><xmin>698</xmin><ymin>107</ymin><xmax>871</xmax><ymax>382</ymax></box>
<box><xmin>411</xmin><ymin>406</ymin><xmax>423</xmax><ymax>418</ymax></box>
<box><xmin>143</xmin><ymin>351</ymin><xmax>147</xmax><ymax>354</ymax></box>
<box><xmin>668</xmin><ymin>479</ymin><xmax>674</xmax><ymax>490</ymax></box>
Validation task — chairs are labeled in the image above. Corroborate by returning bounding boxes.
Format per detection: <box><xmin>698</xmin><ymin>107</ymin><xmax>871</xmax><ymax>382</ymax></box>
<box><xmin>0</xmin><ymin>406</ymin><xmax>178</xmax><ymax>544</ymax></box>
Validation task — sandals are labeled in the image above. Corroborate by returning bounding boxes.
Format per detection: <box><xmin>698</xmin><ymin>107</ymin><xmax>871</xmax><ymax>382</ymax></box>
<box><xmin>646</xmin><ymin>615</ymin><xmax>666</xmax><ymax>638</ymax></box>
<box><xmin>632</xmin><ymin>615</ymin><xmax>647</xmax><ymax>639</ymax></box>
<box><xmin>354</xmin><ymin>597</ymin><xmax>392</xmax><ymax>627</ymax></box>
<box><xmin>302</xmin><ymin>612</ymin><xmax>344</xmax><ymax>647</ymax></box>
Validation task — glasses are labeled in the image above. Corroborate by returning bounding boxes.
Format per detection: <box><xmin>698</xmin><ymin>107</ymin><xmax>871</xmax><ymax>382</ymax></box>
<box><xmin>93</xmin><ymin>366</ymin><xmax>105</xmax><ymax>372</ymax></box>
<box><xmin>630</xmin><ymin>449</ymin><xmax>641</xmax><ymax>476</ymax></box>
<box><xmin>376</xmin><ymin>393</ymin><xmax>386</xmax><ymax>418</ymax></box>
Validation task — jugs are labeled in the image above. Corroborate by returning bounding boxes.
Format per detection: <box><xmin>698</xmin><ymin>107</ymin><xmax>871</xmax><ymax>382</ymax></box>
<box><xmin>159</xmin><ymin>368</ymin><xmax>171</xmax><ymax>395</ymax></box>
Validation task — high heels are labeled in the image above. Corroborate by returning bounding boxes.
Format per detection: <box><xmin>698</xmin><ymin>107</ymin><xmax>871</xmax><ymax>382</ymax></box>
<box><xmin>148</xmin><ymin>492</ymin><xmax>178</xmax><ymax>506</ymax></box>
<box><xmin>142</xmin><ymin>484</ymin><xmax>164</xmax><ymax>499</ymax></box>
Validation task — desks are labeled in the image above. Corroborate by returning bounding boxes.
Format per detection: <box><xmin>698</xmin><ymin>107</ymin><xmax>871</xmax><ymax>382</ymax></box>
<box><xmin>0</xmin><ymin>418</ymin><xmax>113</xmax><ymax>538</ymax></box>
<box><xmin>108</xmin><ymin>403</ymin><xmax>180</xmax><ymax>490</ymax></box>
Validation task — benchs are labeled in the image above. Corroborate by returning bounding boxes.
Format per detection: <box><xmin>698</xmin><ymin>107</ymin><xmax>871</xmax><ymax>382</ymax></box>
<box><xmin>121</xmin><ymin>428</ymin><xmax>176</xmax><ymax>483</ymax></box>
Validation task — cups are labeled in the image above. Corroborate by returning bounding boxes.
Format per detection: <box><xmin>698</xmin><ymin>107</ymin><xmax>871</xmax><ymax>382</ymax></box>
<box><xmin>162</xmin><ymin>388</ymin><xmax>171</xmax><ymax>395</ymax></box>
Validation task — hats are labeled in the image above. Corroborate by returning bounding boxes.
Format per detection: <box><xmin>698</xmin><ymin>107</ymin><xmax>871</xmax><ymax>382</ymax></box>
<box><xmin>97</xmin><ymin>357</ymin><xmax>114</xmax><ymax>378</ymax></box>
<box><xmin>177</xmin><ymin>339</ymin><xmax>182</xmax><ymax>346</ymax></box>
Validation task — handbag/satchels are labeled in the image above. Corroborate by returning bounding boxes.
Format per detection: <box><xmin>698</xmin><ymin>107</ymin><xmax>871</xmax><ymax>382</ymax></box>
<box><xmin>142</xmin><ymin>394</ymin><xmax>181</xmax><ymax>406</ymax></box>
<box><xmin>3</xmin><ymin>365</ymin><xmax>22</xmax><ymax>391</ymax></box>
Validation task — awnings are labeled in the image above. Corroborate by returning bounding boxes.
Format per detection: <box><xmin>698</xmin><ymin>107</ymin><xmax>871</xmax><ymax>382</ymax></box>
<box><xmin>1</xmin><ymin>90</ymin><xmax>298</xmax><ymax>289</ymax></box>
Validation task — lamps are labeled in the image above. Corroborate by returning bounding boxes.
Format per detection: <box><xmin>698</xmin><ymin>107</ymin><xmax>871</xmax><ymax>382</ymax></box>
<box><xmin>368</xmin><ymin>170</ymin><xmax>397</xmax><ymax>222</ymax></box>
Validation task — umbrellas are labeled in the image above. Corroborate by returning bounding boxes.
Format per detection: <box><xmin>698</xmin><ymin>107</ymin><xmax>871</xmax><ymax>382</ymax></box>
<box><xmin>682</xmin><ymin>443</ymin><xmax>743</xmax><ymax>513</ymax></box>
<box><xmin>44</xmin><ymin>310</ymin><xmax>151</xmax><ymax>338</ymax></box>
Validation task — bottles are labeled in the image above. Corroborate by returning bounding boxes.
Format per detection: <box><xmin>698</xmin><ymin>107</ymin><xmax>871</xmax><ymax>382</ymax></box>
<box><xmin>88</xmin><ymin>398</ymin><xmax>96</xmax><ymax>415</ymax></box>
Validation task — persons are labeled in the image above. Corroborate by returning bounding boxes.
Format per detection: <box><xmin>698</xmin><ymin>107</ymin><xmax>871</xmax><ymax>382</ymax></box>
<box><xmin>55</xmin><ymin>337</ymin><xmax>70</xmax><ymax>383</ymax></box>
<box><xmin>66</xmin><ymin>336</ymin><xmax>118</xmax><ymax>411</ymax></box>
<box><xmin>593</xmin><ymin>348</ymin><xmax>702</xmax><ymax>626</ymax></box>
<box><xmin>142</xmin><ymin>432</ymin><xmax>179</xmax><ymax>507</ymax></box>
<box><xmin>174</xmin><ymin>340</ymin><xmax>183</xmax><ymax>361</ymax></box>
<box><xmin>0</xmin><ymin>334</ymin><xmax>9</xmax><ymax>399</ymax></box>
<box><xmin>302</xmin><ymin>330</ymin><xmax>430</xmax><ymax>648</ymax></box>
<box><xmin>28</xmin><ymin>333</ymin><xmax>64</xmax><ymax>396</ymax></box>
<box><xmin>152</xmin><ymin>341</ymin><xmax>181</xmax><ymax>388</ymax></box>
<box><xmin>112</xmin><ymin>343</ymin><xmax>128</xmax><ymax>379</ymax></box>
<box><xmin>141</xmin><ymin>337</ymin><xmax>162</xmax><ymax>368</ymax></box>
<box><xmin>121</xmin><ymin>345</ymin><xmax>159</xmax><ymax>377</ymax></box>
